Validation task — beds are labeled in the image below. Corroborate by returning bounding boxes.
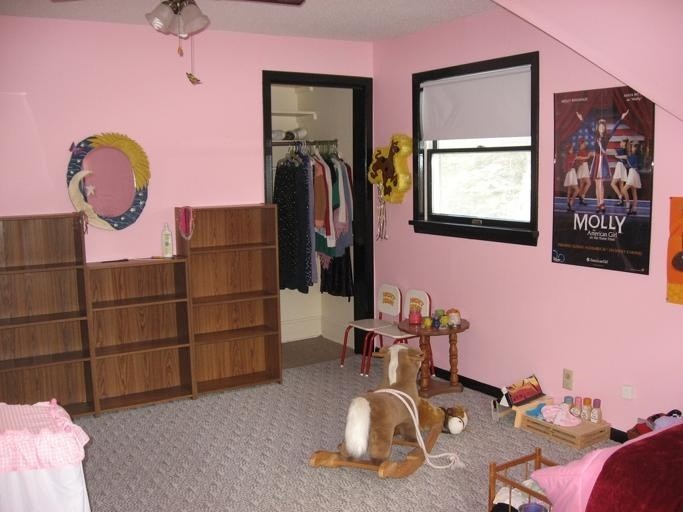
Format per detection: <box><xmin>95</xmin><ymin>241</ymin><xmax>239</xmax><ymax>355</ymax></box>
<box><xmin>530</xmin><ymin>419</ymin><xmax>683</xmax><ymax>512</ymax></box>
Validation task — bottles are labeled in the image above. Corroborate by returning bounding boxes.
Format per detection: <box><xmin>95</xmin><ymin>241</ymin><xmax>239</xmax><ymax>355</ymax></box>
<box><xmin>564</xmin><ymin>396</ymin><xmax>602</xmax><ymax>424</ymax></box>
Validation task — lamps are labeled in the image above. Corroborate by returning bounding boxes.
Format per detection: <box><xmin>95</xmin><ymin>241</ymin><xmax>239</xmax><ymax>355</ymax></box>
<box><xmin>144</xmin><ymin>1</ymin><xmax>210</xmax><ymax>88</ymax></box>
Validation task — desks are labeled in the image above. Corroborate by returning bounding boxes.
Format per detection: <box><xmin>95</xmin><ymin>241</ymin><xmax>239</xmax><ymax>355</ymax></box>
<box><xmin>401</xmin><ymin>317</ymin><xmax>471</xmax><ymax>399</ymax></box>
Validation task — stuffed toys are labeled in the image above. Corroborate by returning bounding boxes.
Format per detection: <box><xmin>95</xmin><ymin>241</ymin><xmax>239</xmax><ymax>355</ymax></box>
<box><xmin>419</xmin><ymin>398</ymin><xmax>468</xmax><ymax>433</ymax></box>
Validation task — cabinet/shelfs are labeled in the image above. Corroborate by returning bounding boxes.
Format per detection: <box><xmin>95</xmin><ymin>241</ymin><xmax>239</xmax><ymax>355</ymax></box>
<box><xmin>2</xmin><ymin>210</ymin><xmax>96</xmax><ymax>417</ymax></box>
<box><xmin>87</xmin><ymin>256</ymin><xmax>195</xmax><ymax>419</ymax></box>
<box><xmin>175</xmin><ymin>203</ymin><xmax>283</xmax><ymax>399</ymax></box>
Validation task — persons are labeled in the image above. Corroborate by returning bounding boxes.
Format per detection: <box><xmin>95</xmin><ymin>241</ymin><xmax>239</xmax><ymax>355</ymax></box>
<box><xmin>611</xmin><ymin>138</ymin><xmax>630</xmax><ymax>210</ymax></box>
<box><xmin>564</xmin><ymin>143</ymin><xmax>590</xmax><ymax>213</ymax></box>
<box><xmin>615</xmin><ymin>144</ymin><xmax>644</xmax><ymax>215</ymax></box>
<box><xmin>575</xmin><ymin>109</ymin><xmax>629</xmax><ymax>213</ymax></box>
<box><xmin>577</xmin><ymin>138</ymin><xmax>596</xmax><ymax>205</ymax></box>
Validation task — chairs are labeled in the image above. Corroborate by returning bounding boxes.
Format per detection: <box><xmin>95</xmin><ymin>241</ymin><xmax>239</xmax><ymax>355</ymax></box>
<box><xmin>366</xmin><ymin>289</ymin><xmax>435</xmax><ymax>378</ymax></box>
<box><xmin>341</xmin><ymin>284</ymin><xmax>402</xmax><ymax>376</ymax></box>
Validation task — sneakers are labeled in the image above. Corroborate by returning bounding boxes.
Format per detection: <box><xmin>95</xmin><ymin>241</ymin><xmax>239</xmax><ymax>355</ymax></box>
<box><xmin>576</xmin><ymin>194</ymin><xmax>584</xmax><ymax>201</ymax></box>
<box><xmin>579</xmin><ymin>199</ymin><xmax>588</xmax><ymax>206</ymax></box>
<box><xmin>625</xmin><ymin>203</ymin><xmax>632</xmax><ymax>215</ymax></box>
<box><xmin>614</xmin><ymin>199</ymin><xmax>622</xmax><ymax>205</ymax></box>
<box><xmin>570</xmin><ymin>206</ymin><xmax>575</xmax><ymax>213</ymax></box>
<box><xmin>601</xmin><ymin>207</ymin><xmax>606</xmax><ymax>211</ymax></box>
<box><xmin>618</xmin><ymin>193</ymin><xmax>624</xmax><ymax>206</ymax></box>
<box><xmin>567</xmin><ymin>206</ymin><xmax>570</xmax><ymax>212</ymax></box>
<box><xmin>631</xmin><ymin>210</ymin><xmax>636</xmax><ymax>214</ymax></box>
<box><xmin>596</xmin><ymin>207</ymin><xmax>599</xmax><ymax>212</ymax></box>
